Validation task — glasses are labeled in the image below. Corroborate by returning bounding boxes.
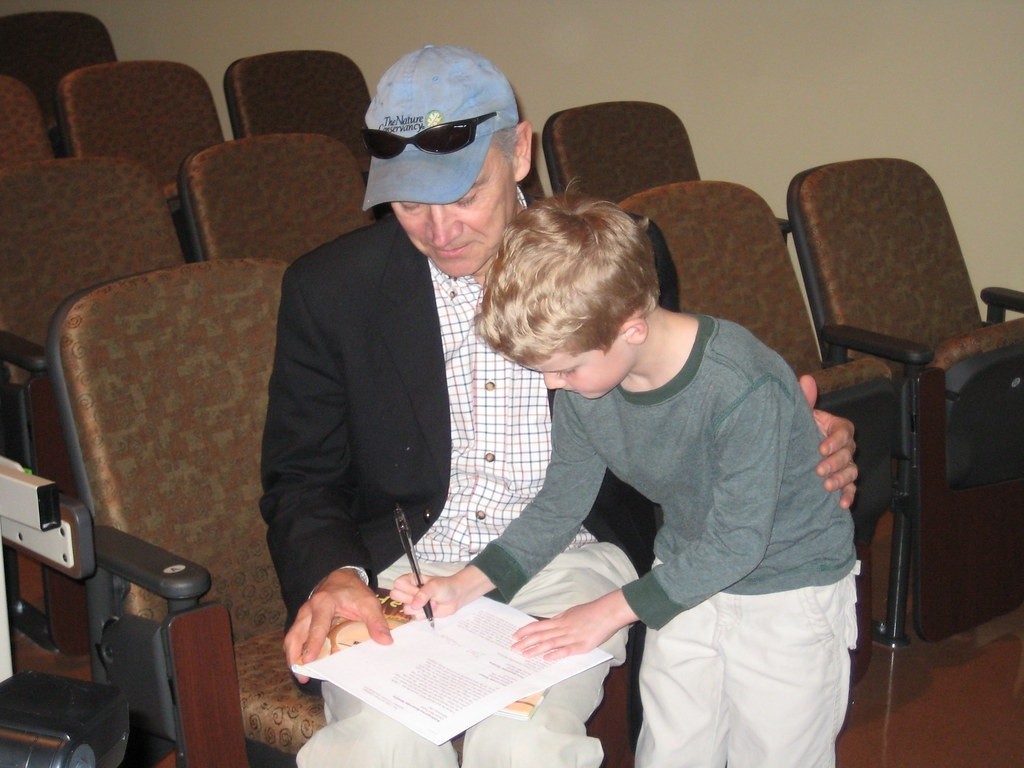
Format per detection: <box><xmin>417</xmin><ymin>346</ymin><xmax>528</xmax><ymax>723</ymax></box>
<box><xmin>362</xmin><ymin>111</ymin><xmax>497</xmax><ymax>160</ymax></box>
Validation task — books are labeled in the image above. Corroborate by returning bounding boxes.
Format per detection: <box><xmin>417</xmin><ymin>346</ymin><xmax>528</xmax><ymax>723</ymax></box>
<box><xmin>289</xmin><ymin>586</ymin><xmax>560</xmax><ymax>723</ymax></box>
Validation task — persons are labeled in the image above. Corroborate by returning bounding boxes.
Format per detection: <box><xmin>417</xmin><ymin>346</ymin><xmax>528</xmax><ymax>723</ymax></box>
<box><xmin>258</xmin><ymin>43</ymin><xmax>859</xmax><ymax>767</ymax></box>
<box><xmin>389</xmin><ymin>193</ymin><xmax>861</xmax><ymax>768</ymax></box>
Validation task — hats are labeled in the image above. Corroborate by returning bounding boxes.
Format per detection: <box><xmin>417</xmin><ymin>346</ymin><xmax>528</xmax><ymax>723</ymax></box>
<box><xmin>362</xmin><ymin>44</ymin><xmax>517</xmax><ymax>213</ymax></box>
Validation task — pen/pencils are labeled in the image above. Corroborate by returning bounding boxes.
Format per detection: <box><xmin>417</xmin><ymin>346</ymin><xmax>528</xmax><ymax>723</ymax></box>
<box><xmin>393</xmin><ymin>505</ymin><xmax>435</xmax><ymax>630</ymax></box>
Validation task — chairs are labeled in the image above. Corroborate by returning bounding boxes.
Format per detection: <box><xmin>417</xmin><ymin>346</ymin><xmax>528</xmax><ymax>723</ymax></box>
<box><xmin>0</xmin><ymin>12</ymin><xmax>1024</xmax><ymax>768</ymax></box>
